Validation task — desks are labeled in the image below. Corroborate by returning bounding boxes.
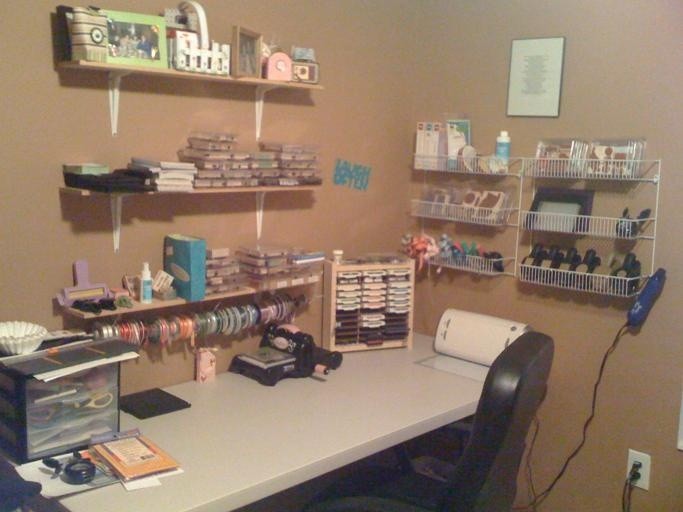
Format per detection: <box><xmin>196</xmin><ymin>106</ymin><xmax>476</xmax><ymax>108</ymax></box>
<box><xmin>15</xmin><ymin>332</ymin><xmax>493</xmax><ymax>512</ymax></box>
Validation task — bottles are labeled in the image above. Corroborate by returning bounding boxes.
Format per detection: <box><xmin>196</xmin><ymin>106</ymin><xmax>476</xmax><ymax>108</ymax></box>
<box><xmin>495</xmin><ymin>130</ymin><xmax>510</xmax><ymax>172</ymax></box>
<box><xmin>139</xmin><ymin>262</ymin><xmax>152</xmax><ymax>304</ymax></box>
<box><xmin>259</xmin><ymin>326</ymin><xmax>341</xmax><ymax>376</ymax></box>
<box><xmin>520</xmin><ymin>242</ymin><xmax>640</xmax><ymax>293</ymax></box>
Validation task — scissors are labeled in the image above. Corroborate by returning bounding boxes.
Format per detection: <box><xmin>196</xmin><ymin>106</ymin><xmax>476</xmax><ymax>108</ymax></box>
<box><xmin>25</xmin><ymin>389</ymin><xmax>114</xmax><ymax>423</ymax></box>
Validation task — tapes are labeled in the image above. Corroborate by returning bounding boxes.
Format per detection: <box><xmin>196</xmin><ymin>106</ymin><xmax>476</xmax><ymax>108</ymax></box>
<box><xmin>65</xmin><ymin>460</ymin><xmax>96</xmax><ymax>484</ymax></box>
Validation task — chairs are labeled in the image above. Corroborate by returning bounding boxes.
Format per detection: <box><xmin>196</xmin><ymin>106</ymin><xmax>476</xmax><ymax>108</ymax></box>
<box><xmin>301</xmin><ymin>331</ymin><xmax>553</xmax><ymax>512</ymax></box>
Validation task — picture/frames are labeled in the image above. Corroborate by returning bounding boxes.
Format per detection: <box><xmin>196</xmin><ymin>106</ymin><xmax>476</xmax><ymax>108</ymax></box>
<box><xmin>505</xmin><ymin>36</ymin><xmax>565</xmax><ymax>118</ymax></box>
<box><xmin>231</xmin><ymin>24</ymin><xmax>260</xmax><ymax>77</ymax></box>
<box><xmin>100</xmin><ymin>10</ymin><xmax>169</xmax><ymax>67</ymax></box>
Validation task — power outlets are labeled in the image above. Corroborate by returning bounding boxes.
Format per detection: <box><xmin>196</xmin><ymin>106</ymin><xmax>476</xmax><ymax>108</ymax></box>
<box><xmin>627</xmin><ymin>448</ymin><xmax>650</xmax><ymax>492</ymax></box>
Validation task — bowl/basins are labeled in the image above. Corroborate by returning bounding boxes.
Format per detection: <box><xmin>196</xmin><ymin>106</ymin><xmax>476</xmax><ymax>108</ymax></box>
<box><xmin>0</xmin><ymin>320</ymin><xmax>46</xmax><ymax>357</ymax></box>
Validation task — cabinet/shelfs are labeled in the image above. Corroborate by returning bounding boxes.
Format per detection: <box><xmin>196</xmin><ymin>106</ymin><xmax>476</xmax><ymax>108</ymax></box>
<box><xmin>407</xmin><ymin>153</ymin><xmax>524</xmax><ymax>277</ymax></box>
<box><xmin>53</xmin><ymin>57</ymin><xmax>327</xmax><ymax>325</ymax></box>
<box><xmin>521</xmin><ymin>158</ymin><xmax>661</xmax><ymax>298</ymax></box>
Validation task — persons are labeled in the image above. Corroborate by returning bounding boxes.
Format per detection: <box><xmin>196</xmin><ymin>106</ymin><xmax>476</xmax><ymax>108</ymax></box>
<box><xmin>242</xmin><ymin>40</ymin><xmax>256</xmax><ymax>75</ymax></box>
<box><xmin>119</xmin><ymin>30</ymin><xmax>151</xmax><ymax>58</ymax></box>
